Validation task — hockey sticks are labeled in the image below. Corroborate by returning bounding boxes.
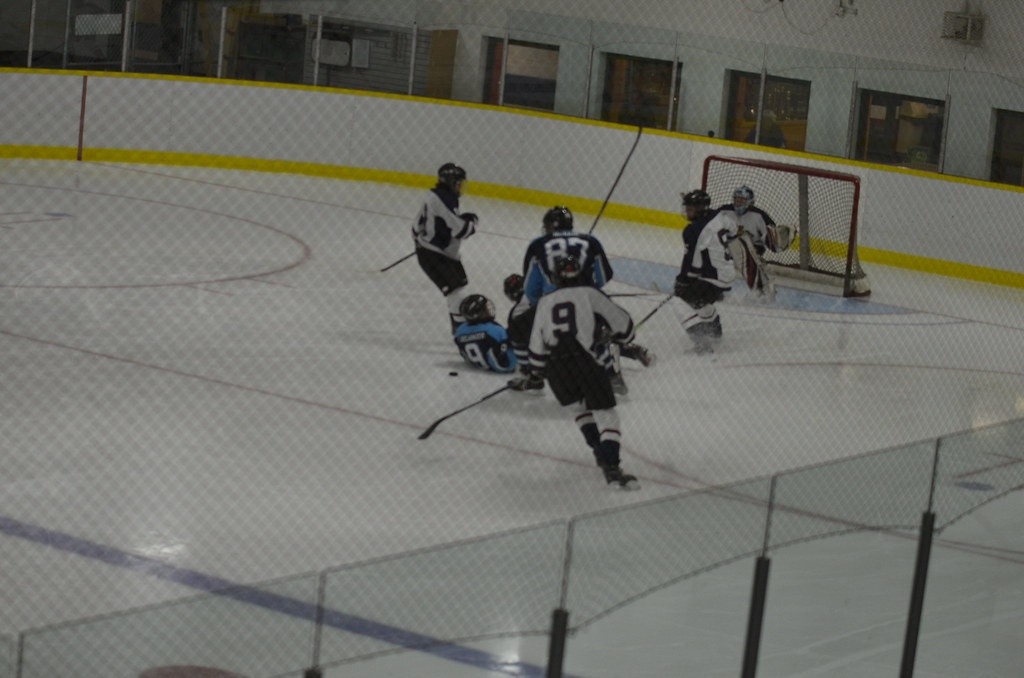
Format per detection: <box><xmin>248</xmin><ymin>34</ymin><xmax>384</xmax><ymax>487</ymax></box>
<box><xmin>417</xmin><ymin>385</ymin><xmax>511</xmax><ymax>440</ymax></box>
<box><xmin>588</xmin><ymin>112</ymin><xmax>643</xmax><ymax>235</ymax></box>
<box><xmin>635</xmin><ymin>292</ymin><xmax>676</xmax><ymax>332</ymax></box>
<box><xmin>606</xmin><ymin>281</ymin><xmax>664</xmax><ymax>298</ymax></box>
<box><xmin>379</xmin><ymin>251</ymin><xmax>417</xmax><ymax>273</ymax></box>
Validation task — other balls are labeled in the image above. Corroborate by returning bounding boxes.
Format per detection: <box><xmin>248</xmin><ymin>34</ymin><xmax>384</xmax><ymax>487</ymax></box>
<box><xmin>449</xmin><ymin>371</ymin><xmax>458</xmax><ymax>376</ymax></box>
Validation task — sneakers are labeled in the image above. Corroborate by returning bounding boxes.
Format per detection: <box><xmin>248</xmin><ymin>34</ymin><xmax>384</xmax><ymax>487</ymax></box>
<box><xmin>617</xmin><ymin>473</ymin><xmax>641</xmax><ymax>489</ymax></box>
<box><xmin>707</xmin><ymin>315</ymin><xmax>722</xmax><ymax>340</ymax></box>
<box><xmin>630</xmin><ymin>344</ymin><xmax>655</xmax><ymax>367</ymax></box>
<box><xmin>683</xmin><ymin>341</ymin><xmax>713</xmax><ymax>358</ymax></box>
<box><xmin>606</xmin><ymin>371</ymin><xmax>629</xmax><ymax>394</ymax></box>
<box><xmin>605</xmin><ymin>466</ymin><xmax>622</xmax><ymax>487</ymax></box>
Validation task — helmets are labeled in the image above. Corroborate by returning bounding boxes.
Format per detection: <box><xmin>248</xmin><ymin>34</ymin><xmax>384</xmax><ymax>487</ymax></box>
<box><xmin>503</xmin><ymin>274</ymin><xmax>525</xmax><ymax>303</ymax></box>
<box><xmin>459</xmin><ymin>294</ymin><xmax>494</xmax><ymax>325</ymax></box>
<box><xmin>681</xmin><ymin>190</ymin><xmax>712</xmax><ymax>222</ymax></box>
<box><xmin>543</xmin><ymin>206</ymin><xmax>573</xmax><ymax>236</ymax></box>
<box><xmin>731</xmin><ymin>186</ymin><xmax>753</xmax><ymax>216</ymax></box>
<box><xmin>437</xmin><ymin>163</ymin><xmax>466</xmax><ymax>191</ymax></box>
<box><xmin>552</xmin><ymin>256</ymin><xmax>583</xmax><ymax>286</ymax></box>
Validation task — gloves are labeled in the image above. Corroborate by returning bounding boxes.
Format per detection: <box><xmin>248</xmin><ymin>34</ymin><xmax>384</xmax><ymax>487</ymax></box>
<box><xmin>674</xmin><ymin>275</ymin><xmax>698</xmax><ymax>299</ymax></box>
<box><xmin>528</xmin><ymin>370</ymin><xmax>545</xmax><ymax>390</ymax></box>
<box><xmin>459</xmin><ymin>212</ymin><xmax>479</xmax><ymax>224</ymax></box>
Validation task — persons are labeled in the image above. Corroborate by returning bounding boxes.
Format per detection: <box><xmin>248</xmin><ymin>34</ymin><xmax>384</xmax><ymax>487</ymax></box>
<box><xmin>672</xmin><ymin>185</ymin><xmax>798</xmax><ymax>354</ymax></box>
<box><xmin>412</xmin><ymin>162</ymin><xmax>656</xmax><ymax>490</ymax></box>
